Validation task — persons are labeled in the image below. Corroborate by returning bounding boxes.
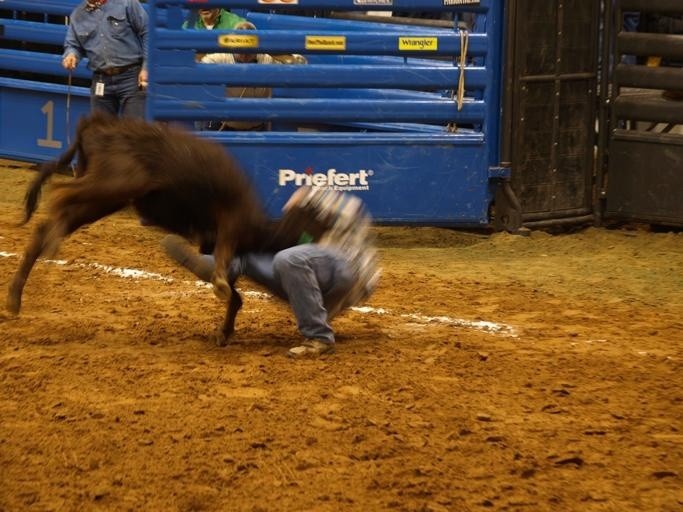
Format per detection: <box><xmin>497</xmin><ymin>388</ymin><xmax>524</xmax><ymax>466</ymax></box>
<box><xmin>161</xmin><ymin>187</ymin><xmax>382</xmax><ymax>359</ymax></box>
<box><xmin>181</xmin><ymin>8</ymin><xmax>246</xmax><ymax>30</ymax></box>
<box><xmin>198</xmin><ymin>22</ymin><xmax>308</xmax><ymax>132</ymax></box>
<box><xmin>63</xmin><ymin>0</ymin><xmax>149</xmax><ymax>118</ymax></box>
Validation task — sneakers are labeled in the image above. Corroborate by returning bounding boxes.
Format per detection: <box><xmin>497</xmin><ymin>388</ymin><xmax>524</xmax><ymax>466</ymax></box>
<box><xmin>288</xmin><ymin>340</ymin><xmax>334</xmax><ymax>357</ymax></box>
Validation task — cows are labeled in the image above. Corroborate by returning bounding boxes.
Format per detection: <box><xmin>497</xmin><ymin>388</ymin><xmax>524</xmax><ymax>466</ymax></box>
<box><xmin>3</xmin><ymin>109</ymin><xmax>298</xmax><ymax>349</ymax></box>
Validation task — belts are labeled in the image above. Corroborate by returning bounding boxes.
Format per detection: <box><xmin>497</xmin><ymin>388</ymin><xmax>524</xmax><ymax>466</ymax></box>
<box><xmin>104</xmin><ymin>64</ymin><xmax>134</xmax><ymax>75</ymax></box>
